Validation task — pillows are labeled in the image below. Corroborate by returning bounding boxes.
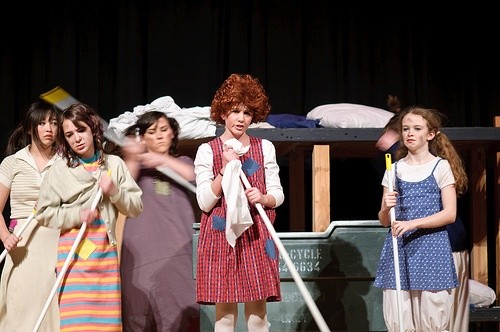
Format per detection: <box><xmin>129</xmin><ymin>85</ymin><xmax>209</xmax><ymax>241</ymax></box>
<box><xmin>306</xmin><ymin>102</ymin><xmax>395</xmax><ymax>128</ymax></box>
<box><xmin>468</xmin><ymin>279</ymin><xmax>496</xmax><ymax>307</ymax></box>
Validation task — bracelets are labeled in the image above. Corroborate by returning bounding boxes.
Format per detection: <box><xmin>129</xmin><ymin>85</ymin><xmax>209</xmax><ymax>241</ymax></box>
<box><xmin>219</xmin><ymin>171</ymin><xmax>224</xmax><ymax>177</ymax></box>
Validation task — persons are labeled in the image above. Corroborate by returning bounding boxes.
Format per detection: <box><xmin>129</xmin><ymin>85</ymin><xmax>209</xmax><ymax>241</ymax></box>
<box><xmin>372</xmin><ymin>108</ymin><xmax>469</xmax><ymax>332</ymax></box>
<box><xmin>193</xmin><ymin>72</ymin><xmax>286</xmax><ymax>332</ymax></box>
<box><xmin>113</xmin><ymin>111</ymin><xmax>200</xmax><ymax>332</ymax></box>
<box><xmin>36</xmin><ymin>103</ymin><xmax>144</xmax><ymax>332</ymax></box>
<box><xmin>0</xmin><ymin>101</ymin><xmax>66</xmax><ymax>332</ymax></box>
